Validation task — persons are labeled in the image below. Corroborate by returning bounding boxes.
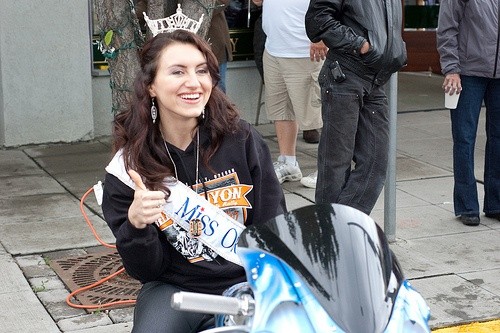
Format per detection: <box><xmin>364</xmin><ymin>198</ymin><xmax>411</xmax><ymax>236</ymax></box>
<box><xmin>206</xmin><ymin>0</ymin><xmax>234</xmax><ymax>94</ymax></box>
<box><xmin>436</xmin><ymin>0</ymin><xmax>500</xmax><ymax>225</ymax></box>
<box><xmin>101</xmin><ymin>28</ymin><xmax>288</xmax><ymax>333</ymax></box>
<box><xmin>253</xmin><ymin>15</ymin><xmax>320</xmax><ymax>143</ymax></box>
<box><xmin>252</xmin><ymin>0</ymin><xmax>329</xmax><ymax>190</ymax></box>
<box><xmin>305</xmin><ymin>0</ymin><xmax>408</xmax><ymax>215</ymax></box>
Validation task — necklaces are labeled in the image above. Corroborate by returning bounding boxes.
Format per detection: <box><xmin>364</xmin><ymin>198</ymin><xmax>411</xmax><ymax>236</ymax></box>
<box><xmin>158</xmin><ymin>121</ymin><xmax>202</xmax><ymax>237</ymax></box>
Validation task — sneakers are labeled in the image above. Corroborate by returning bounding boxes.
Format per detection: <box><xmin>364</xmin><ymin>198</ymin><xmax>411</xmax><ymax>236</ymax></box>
<box><xmin>273</xmin><ymin>155</ymin><xmax>302</xmax><ymax>185</ymax></box>
<box><xmin>301</xmin><ymin>170</ymin><xmax>318</xmax><ymax>189</ymax></box>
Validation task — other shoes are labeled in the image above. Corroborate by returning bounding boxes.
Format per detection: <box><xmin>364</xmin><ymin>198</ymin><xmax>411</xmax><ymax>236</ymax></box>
<box><xmin>305</xmin><ymin>129</ymin><xmax>319</xmax><ymax>143</ymax></box>
<box><xmin>486</xmin><ymin>212</ymin><xmax>500</xmax><ymax>221</ymax></box>
<box><xmin>461</xmin><ymin>214</ymin><xmax>479</xmax><ymax>225</ymax></box>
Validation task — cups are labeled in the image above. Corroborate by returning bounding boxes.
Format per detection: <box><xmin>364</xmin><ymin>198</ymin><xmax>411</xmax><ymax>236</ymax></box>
<box><xmin>444</xmin><ymin>87</ymin><xmax>462</xmax><ymax>110</ymax></box>
